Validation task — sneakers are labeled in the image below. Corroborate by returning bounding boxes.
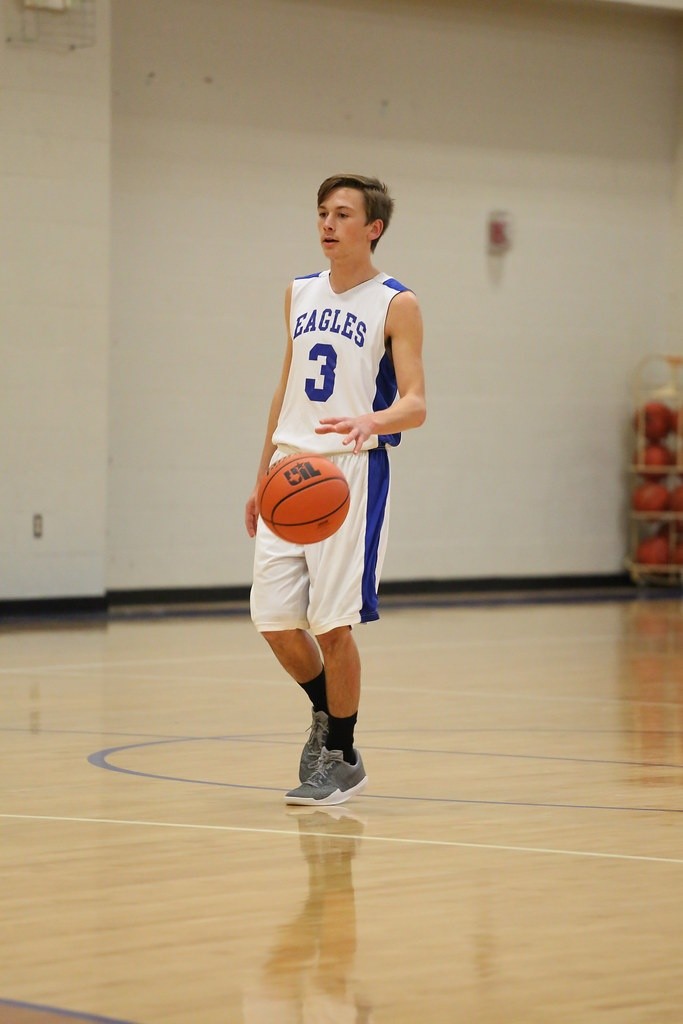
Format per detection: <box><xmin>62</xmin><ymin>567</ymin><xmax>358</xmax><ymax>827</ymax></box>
<box><xmin>299</xmin><ymin>706</ymin><xmax>328</xmax><ymax>785</ymax></box>
<box><xmin>284</xmin><ymin>748</ymin><xmax>367</xmax><ymax>805</ymax></box>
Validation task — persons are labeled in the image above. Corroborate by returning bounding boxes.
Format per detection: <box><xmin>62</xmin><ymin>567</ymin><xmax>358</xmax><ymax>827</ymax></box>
<box><xmin>244</xmin><ymin>174</ymin><xmax>427</xmax><ymax>806</ymax></box>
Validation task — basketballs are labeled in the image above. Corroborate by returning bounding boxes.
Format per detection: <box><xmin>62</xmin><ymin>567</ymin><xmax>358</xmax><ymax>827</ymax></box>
<box><xmin>254</xmin><ymin>448</ymin><xmax>352</xmax><ymax>545</ymax></box>
<box><xmin>631</xmin><ymin>400</ymin><xmax>683</xmax><ymax>566</ymax></box>
